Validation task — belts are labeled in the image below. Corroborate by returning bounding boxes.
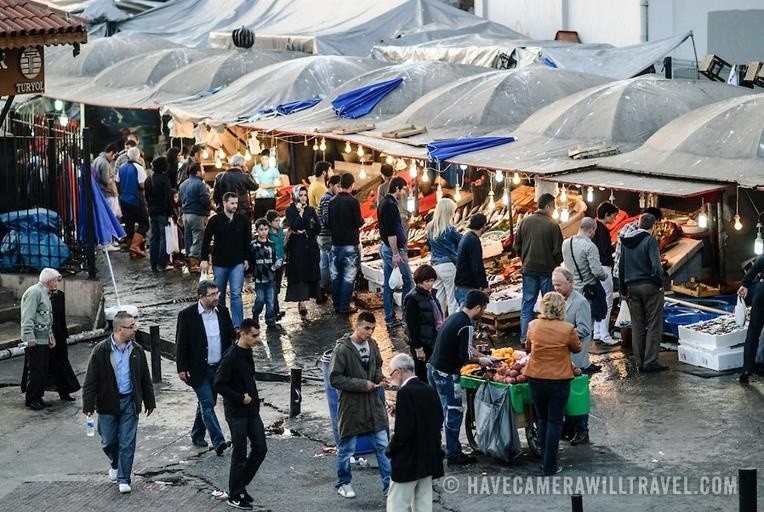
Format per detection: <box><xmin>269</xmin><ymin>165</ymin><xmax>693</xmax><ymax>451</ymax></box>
<box><xmin>119</xmin><ymin>391</ymin><xmax>134</xmax><ymax>398</ymax></box>
<box><xmin>208</xmin><ymin>363</ymin><xmax>220</xmax><ymax>367</ymax></box>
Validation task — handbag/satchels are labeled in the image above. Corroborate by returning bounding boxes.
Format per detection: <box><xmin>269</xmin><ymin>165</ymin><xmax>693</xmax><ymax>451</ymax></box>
<box><xmin>165</xmin><ymin>217</ymin><xmax>180</xmax><ymax>255</ymax></box>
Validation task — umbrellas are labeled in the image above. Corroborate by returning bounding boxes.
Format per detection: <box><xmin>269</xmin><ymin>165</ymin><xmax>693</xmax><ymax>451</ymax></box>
<box><xmin>331</xmin><ymin>75</ymin><xmax>408</xmax><ymax>120</ymax></box>
<box><xmin>78</xmin><ymin>164</ymin><xmax>127</xmax><ymax>308</ymax></box>
<box><xmin>233</xmin><ymin>94</ymin><xmax>326</xmax><ymax>123</ymax></box>
<box><xmin>425</xmin><ymin>136</ymin><xmax>519</xmax><ymax>163</ymax></box>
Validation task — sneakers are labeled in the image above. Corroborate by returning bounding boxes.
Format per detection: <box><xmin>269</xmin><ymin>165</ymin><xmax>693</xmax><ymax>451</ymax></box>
<box><xmin>239</xmin><ymin>493</ymin><xmax>253</xmax><ymax>502</ymax></box>
<box><xmin>119</xmin><ymin>484</ymin><xmax>131</xmax><ymax>492</ymax></box>
<box><xmin>253</xmin><ymin>313</ymin><xmax>259</xmax><ymax>322</ymax></box>
<box><xmin>108</xmin><ymin>467</ymin><xmax>118</xmax><ymax>481</ymax></box>
<box><xmin>103</xmin><ymin>245</ymin><xmax>121</xmax><ymax>252</ymax></box>
<box><xmin>227</xmin><ymin>499</ymin><xmax>252</xmax><ymax>509</ymax></box>
<box><xmin>234</xmin><ymin>327</ymin><xmax>240</xmax><ymax>335</ymax></box>
<box><xmin>600</xmin><ymin>337</ymin><xmax>620</xmax><ymax>345</ymax></box>
<box><xmin>276</xmin><ymin>311</ymin><xmax>285</xmax><ymax>322</ymax></box>
<box><xmin>447</xmin><ymin>453</ymin><xmax>477</xmax><ymax>465</ymax></box>
<box><xmin>386</xmin><ymin>319</ymin><xmax>402</xmax><ymax>328</ymax></box>
<box><xmin>268</xmin><ymin>322</ymin><xmax>276</xmax><ymax>328</ymax></box>
<box><xmin>546</xmin><ymin>466</ymin><xmax>562</xmax><ymax>475</ymax></box>
<box><xmin>337</xmin><ymin>484</ymin><xmax>356</xmax><ymax>498</ymax></box>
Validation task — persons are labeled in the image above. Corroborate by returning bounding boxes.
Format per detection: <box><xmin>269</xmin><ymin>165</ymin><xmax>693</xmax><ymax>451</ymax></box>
<box><xmin>426</xmin><ymin>290</ymin><xmax>499</xmax><ymax>466</ymax></box>
<box><xmin>175</xmin><ymin>143</ymin><xmax>204</xmax><ymax>255</ymax></box>
<box><xmin>265</xmin><ymin>209</ymin><xmax>286</xmax><ymax>322</ymax></box>
<box><xmin>377</xmin><ymin>177</ymin><xmax>415</xmax><ymax>329</ymax></box>
<box><xmin>250</xmin><ymin>219</ymin><xmax>282</xmax><ymax>329</ymax></box>
<box><xmin>610</xmin><ymin>205</ymin><xmax>663</xmax><ymax>340</ymax></box>
<box><xmin>14</xmin><ymin>144</ymin><xmax>79</xmax><ymax>211</ymax></box>
<box><xmin>200</xmin><ymin>192</ymin><xmax>253</xmax><ymax>334</ymax></box>
<box><xmin>212</xmin><ymin>155</ymin><xmax>260</xmax><ymax>222</ymax></box>
<box><xmin>113</xmin><ymin>147</ymin><xmax>150</xmax><ymax>258</ymax></box>
<box><xmin>737</xmin><ymin>252</ymin><xmax>764</xmax><ymax>384</ymax></box>
<box><xmin>176</xmin><ymin>161</ymin><xmax>217</xmax><ymax>274</ymax></box>
<box><xmin>145</xmin><ymin>157</ymin><xmax>175</xmax><ymax>272</ymax></box>
<box><xmin>513</xmin><ymin>193</ymin><xmax>564</xmax><ymax>348</ymax></box>
<box><xmin>454</xmin><ymin>213</ymin><xmax>491</xmax><ymax>311</ymax></box>
<box><xmin>524</xmin><ymin>292</ymin><xmax>583</xmax><ymax>476</ymax></box>
<box><xmin>551</xmin><ymin>267</ymin><xmax>593</xmax><ymax>444</ymax></box>
<box><xmin>469</xmin><ymin>167</ymin><xmax>507</xmax><ymax>211</ymax></box>
<box><xmin>213</xmin><ymin>318</ymin><xmax>268</xmax><ymax>510</ymax></box>
<box><xmin>175</xmin><ymin>279</ymin><xmax>238</xmax><ymax>456</ymax></box>
<box><xmin>377</xmin><ymin>164</ymin><xmax>411</xmax><ymax>241</ymax></box>
<box><xmin>403</xmin><ymin>264</ymin><xmax>446</xmax><ymax>383</ymax></box>
<box><xmin>593</xmin><ymin>201</ymin><xmax>620</xmax><ymax>346</ymax></box>
<box><xmin>329</xmin><ymin>312</ymin><xmax>391</xmax><ymax>497</ymax></box>
<box><xmin>384</xmin><ymin>354</ymin><xmax>446</xmax><ymax>512</ymax></box>
<box><xmin>164</xmin><ymin>191</ymin><xmax>180</xmax><ymax>269</ymax></box>
<box><xmin>617</xmin><ymin>214</ymin><xmax>672</xmax><ymax>372</ymax></box>
<box><xmin>166</xmin><ymin>147</ymin><xmax>179</xmax><ymax>189</ymax></box>
<box><xmin>308</xmin><ymin>162</ymin><xmax>335</xmax><ymax>213</ymax></box>
<box><xmin>116</xmin><ymin>133</ymin><xmax>144</xmax><ymax>159</ymax></box>
<box><xmin>91</xmin><ymin>144</ymin><xmax>121</xmax><ymax>226</ymax></box>
<box><xmin>20</xmin><ymin>271</ymin><xmax>82</xmax><ymax>402</ymax></box>
<box><xmin>317</xmin><ymin>175</ymin><xmax>342</xmax><ymax>303</ymax></box>
<box><xmin>562</xmin><ymin>217</ymin><xmax>609</xmax><ymax>375</ymax></box>
<box><xmin>250</xmin><ymin>149</ymin><xmax>284</xmax><ymax>222</ymax></box>
<box><xmin>329</xmin><ymin>173</ymin><xmax>365</xmax><ymax>315</ymax></box>
<box><xmin>83</xmin><ymin>312</ymin><xmax>156</xmax><ymax>493</ymax></box>
<box><xmin>20</xmin><ymin>267</ymin><xmax>57</xmax><ymax>411</ymax></box>
<box><xmin>283</xmin><ymin>185</ymin><xmax>322</xmax><ymax>315</ymax></box>
<box><xmin>114</xmin><ymin>139</ymin><xmax>150</xmax><ymax>251</ymax></box>
<box><xmin>425</xmin><ymin>198</ymin><xmax>464</xmax><ymax>320</ymax></box>
<box><xmin>424</xmin><ymin>194</ymin><xmax>457</xmax><ymax>251</ymax></box>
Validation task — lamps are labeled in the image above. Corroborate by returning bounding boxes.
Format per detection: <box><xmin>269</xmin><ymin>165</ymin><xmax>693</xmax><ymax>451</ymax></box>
<box><xmin>216</xmin><ymin>158</ymin><xmax>221</xmax><ymax>169</ymax></box>
<box><xmin>409</xmin><ymin>159</ymin><xmax>417</xmax><ymax>178</ymax></box>
<box><xmin>218</xmin><ymin>148</ymin><xmax>226</xmax><ymax>159</ymax></box>
<box><xmin>732</xmin><ymin>186</ymin><xmax>742</xmax><ymax>230</ymax></box>
<box><xmin>406</xmin><ymin>189</ymin><xmax>416</xmax><ymax>212</ymax></box>
<box><xmin>587</xmin><ymin>187</ymin><xmax>593</xmax><ymax>202</ymax></box>
<box><xmin>639</xmin><ymin>193</ymin><xmax>645</xmax><ymax>208</ymax></box>
<box><xmin>698</xmin><ymin>198</ymin><xmax>707</xmax><ymax>228</ymax></box>
<box><xmin>320</xmin><ymin>137</ymin><xmax>326</xmax><ymax>151</ymax></box>
<box><xmin>560</xmin><ymin>210</ymin><xmax>569</xmax><ymax>222</ymax></box>
<box><xmin>503</xmin><ymin>171</ymin><xmax>509</xmax><ymax>206</ymax></box>
<box><xmin>454</xmin><ymin>165</ymin><xmax>463</xmax><ymax>202</ymax></box>
<box><xmin>551</xmin><ymin>208</ymin><xmax>559</xmax><ymax>221</ymax></box>
<box><xmin>385</xmin><ymin>155</ymin><xmax>395</xmax><ymax>166</ymax></box>
<box><xmin>560</xmin><ymin>184</ymin><xmax>567</xmax><ymax>202</ymax></box>
<box><xmin>313</xmin><ymin>139</ymin><xmax>318</xmax><ymax>151</ymax></box>
<box><xmin>435</xmin><ymin>162</ymin><xmax>443</xmax><ymax>201</ymax></box>
<box><xmin>359</xmin><ymin>158</ymin><xmax>366</xmax><ymax>179</ymax></box>
<box><xmin>202</xmin><ymin>147</ymin><xmax>208</xmax><ymax>159</ymax></box>
<box><xmin>489</xmin><ymin>176</ymin><xmax>495</xmax><ymax>209</ymax></box>
<box><xmin>235</xmin><ymin>150</ymin><xmax>243</xmax><ymax>162</ymax></box>
<box><xmin>511</xmin><ymin>169</ymin><xmax>520</xmax><ymax>185</ymax></box>
<box><xmin>422</xmin><ymin>160</ymin><xmax>429</xmax><ymax>183</ymax></box>
<box><xmin>248</xmin><ymin>131</ymin><xmax>261</xmax><ymax>155</ymax></box>
<box><xmin>54</xmin><ymin>99</ymin><xmax>63</xmax><ymax>111</ymax></box>
<box><xmin>396</xmin><ymin>157</ymin><xmax>407</xmax><ymax>172</ymax></box>
<box><xmin>269</xmin><ymin>130</ymin><xmax>276</xmax><ymax>167</ymax></box>
<box><xmin>357</xmin><ymin>144</ymin><xmax>364</xmax><ymax>157</ymax></box>
<box><xmin>495</xmin><ymin>170</ymin><xmax>504</xmax><ymax>182</ymax></box>
<box><xmin>59</xmin><ymin>106</ymin><xmax>68</xmax><ymax>127</ymax></box>
<box><xmin>754</xmin><ymin>211</ymin><xmax>764</xmax><ymax>255</ymax></box>
<box><xmin>345</xmin><ymin>140</ymin><xmax>352</xmax><ymax>153</ymax></box>
<box><xmin>244</xmin><ymin>128</ymin><xmax>251</xmax><ymax>163</ymax></box>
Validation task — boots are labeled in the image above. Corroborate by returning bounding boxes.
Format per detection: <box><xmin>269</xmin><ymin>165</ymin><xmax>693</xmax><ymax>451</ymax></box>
<box><xmin>129</xmin><ymin>233</ymin><xmax>145</xmax><ymax>256</ymax></box>
<box><xmin>189</xmin><ymin>257</ymin><xmax>200</xmax><ymax>272</ymax></box>
<box><xmin>128</xmin><ymin>240</ymin><xmax>138</xmax><ymax>256</ymax></box>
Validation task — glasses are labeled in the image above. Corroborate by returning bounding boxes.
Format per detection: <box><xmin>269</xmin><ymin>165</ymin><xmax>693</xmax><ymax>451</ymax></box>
<box><xmin>206</xmin><ymin>292</ymin><xmax>221</xmax><ymax>297</ymax></box>
<box><xmin>120</xmin><ymin>325</ymin><xmax>137</xmax><ymax>330</ymax></box>
<box><xmin>390</xmin><ymin>368</ymin><xmax>401</xmax><ymax>378</ymax></box>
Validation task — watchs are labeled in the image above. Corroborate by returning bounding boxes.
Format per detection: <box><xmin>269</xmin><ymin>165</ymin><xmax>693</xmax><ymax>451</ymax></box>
<box><xmin>392</xmin><ymin>253</ymin><xmax>400</xmax><ymax>258</ymax></box>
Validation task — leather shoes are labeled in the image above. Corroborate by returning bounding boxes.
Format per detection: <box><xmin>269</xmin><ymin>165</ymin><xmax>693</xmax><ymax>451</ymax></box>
<box><xmin>570</xmin><ymin>431</ymin><xmax>589</xmax><ymax>446</ymax></box>
<box><xmin>583</xmin><ymin>364</ymin><xmax>602</xmax><ymax>373</ymax></box>
<box><xmin>195</xmin><ymin>440</ymin><xmax>208</xmax><ymax>446</ymax></box>
<box><xmin>350</xmin><ymin>307</ymin><xmax>358</xmax><ymax>313</ymax></box>
<box><xmin>40</xmin><ymin>401</ymin><xmax>53</xmax><ymax>407</ymax></box>
<box><xmin>215</xmin><ymin>441</ymin><xmax>227</xmax><ymax>456</ymax></box>
<box><xmin>740</xmin><ymin>373</ymin><xmax>749</xmax><ymax>383</ymax></box>
<box><xmin>25</xmin><ymin>402</ymin><xmax>45</xmax><ymax>410</ymax></box>
<box><xmin>299</xmin><ymin>308</ymin><xmax>307</xmax><ymax>316</ymax></box>
<box><xmin>60</xmin><ymin>396</ymin><xmax>76</xmax><ymax>401</ymax></box>
<box><xmin>649</xmin><ymin>365</ymin><xmax>669</xmax><ymax>372</ymax></box>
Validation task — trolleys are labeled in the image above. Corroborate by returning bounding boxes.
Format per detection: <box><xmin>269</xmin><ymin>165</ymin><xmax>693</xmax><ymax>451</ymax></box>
<box><xmin>460</xmin><ymin>374</ymin><xmax>590</xmax><ymax>456</ymax></box>
